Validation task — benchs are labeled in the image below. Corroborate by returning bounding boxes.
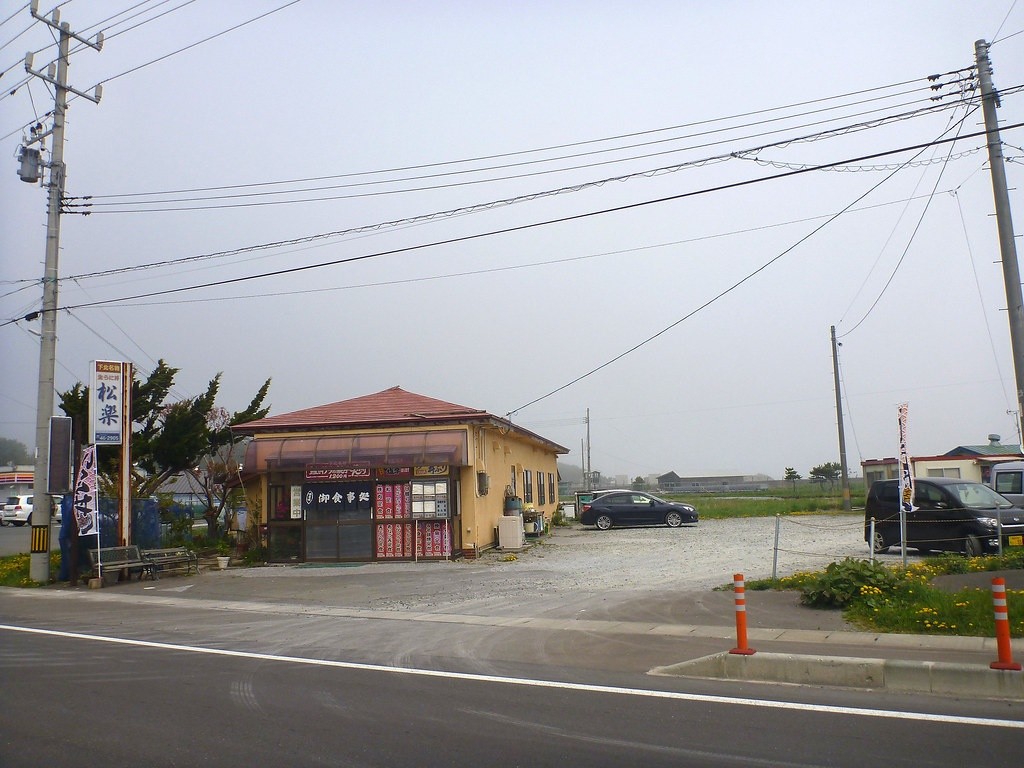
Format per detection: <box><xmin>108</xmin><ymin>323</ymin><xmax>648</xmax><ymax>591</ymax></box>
<box><xmin>87</xmin><ymin>543</ymin><xmax>154</xmax><ymax>583</ymax></box>
<box><xmin>139</xmin><ymin>546</ymin><xmax>201</xmax><ymax>580</ymax></box>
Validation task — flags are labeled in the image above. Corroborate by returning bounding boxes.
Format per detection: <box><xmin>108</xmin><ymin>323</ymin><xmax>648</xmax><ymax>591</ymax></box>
<box><xmin>73</xmin><ymin>445</ymin><xmax>96</xmax><ymax>536</ymax></box>
<box><xmin>898</xmin><ymin>401</ymin><xmax>919</xmax><ymax>513</ymax></box>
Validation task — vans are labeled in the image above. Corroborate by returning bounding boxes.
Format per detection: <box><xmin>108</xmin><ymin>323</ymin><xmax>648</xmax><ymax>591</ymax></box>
<box><xmin>864</xmin><ymin>476</ymin><xmax>1024</xmax><ymax>561</ymax></box>
<box><xmin>990</xmin><ymin>460</ymin><xmax>1024</xmax><ymax>511</ymax></box>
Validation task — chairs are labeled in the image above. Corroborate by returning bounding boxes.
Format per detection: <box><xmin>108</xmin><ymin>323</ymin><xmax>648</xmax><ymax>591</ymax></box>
<box><xmin>939</xmin><ymin>491</ymin><xmax>950</xmax><ymax>501</ymax></box>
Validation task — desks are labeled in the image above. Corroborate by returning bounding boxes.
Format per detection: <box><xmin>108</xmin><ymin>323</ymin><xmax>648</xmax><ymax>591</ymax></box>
<box><xmin>522</xmin><ymin>510</ymin><xmax>544</xmax><ymax>538</ymax></box>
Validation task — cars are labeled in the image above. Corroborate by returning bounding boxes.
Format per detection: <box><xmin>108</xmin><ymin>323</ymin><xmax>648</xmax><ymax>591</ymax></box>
<box><xmin>0</xmin><ymin>495</ymin><xmax>64</xmax><ymax>527</ymax></box>
<box><xmin>580</xmin><ymin>491</ymin><xmax>700</xmax><ymax>530</ymax></box>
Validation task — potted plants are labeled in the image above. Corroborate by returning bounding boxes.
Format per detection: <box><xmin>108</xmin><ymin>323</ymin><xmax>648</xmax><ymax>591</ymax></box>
<box><xmin>216</xmin><ymin>537</ymin><xmax>231</xmax><ymax>568</ymax></box>
<box><xmin>241</xmin><ymin>543</ymin><xmax>265</xmax><ymax>566</ymax></box>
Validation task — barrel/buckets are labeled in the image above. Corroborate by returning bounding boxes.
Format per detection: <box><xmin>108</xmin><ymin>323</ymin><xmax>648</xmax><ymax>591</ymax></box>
<box><xmin>524</xmin><ymin>523</ymin><xmax>534</xmax><ymax>532</ymax></box>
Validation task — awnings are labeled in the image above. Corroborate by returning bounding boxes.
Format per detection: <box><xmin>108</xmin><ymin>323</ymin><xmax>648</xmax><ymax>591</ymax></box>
<box><xmin>242</xmin><ymin>429</ymin><xmax>467</xmax><ymax>473</ymax></box>
